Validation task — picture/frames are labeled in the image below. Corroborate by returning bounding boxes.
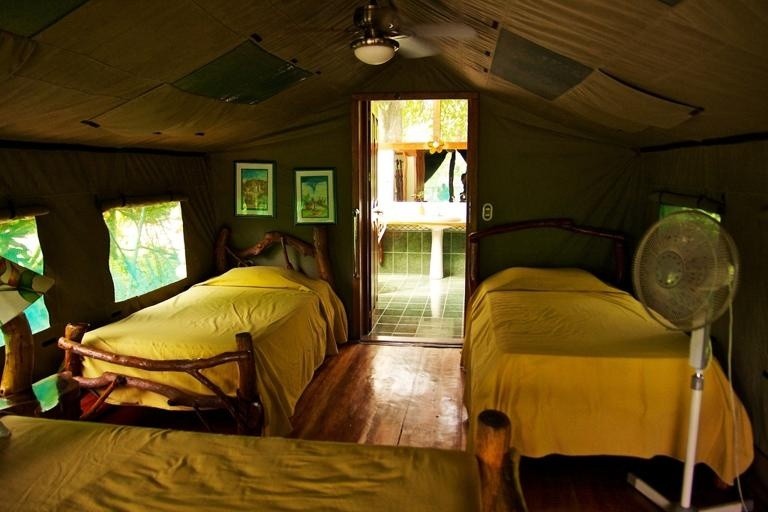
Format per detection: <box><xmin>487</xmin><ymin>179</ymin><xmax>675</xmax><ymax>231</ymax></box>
<box><xmin>236</xmin><ymin>161</ymin><xmax>272</xmax><ymax>216</ymax></box>
<box><xmin>294</xmin><ymin>168</ymin><xmax>335</xmax><ymax>224</ymax></box>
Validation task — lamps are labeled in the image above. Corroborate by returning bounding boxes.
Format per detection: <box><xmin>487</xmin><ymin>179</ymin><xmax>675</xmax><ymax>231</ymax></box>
<box><xmin>351</xmin><ymin>4</ymin><xmax>399</xmax><ymax>67</ymax></box>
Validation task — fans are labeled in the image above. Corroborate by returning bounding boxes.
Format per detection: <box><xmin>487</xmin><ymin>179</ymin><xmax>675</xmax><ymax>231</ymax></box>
<box><xmin>634</xmin><ymin>213</ymin><xmax>740</xmax><ymax>512</ymax></box>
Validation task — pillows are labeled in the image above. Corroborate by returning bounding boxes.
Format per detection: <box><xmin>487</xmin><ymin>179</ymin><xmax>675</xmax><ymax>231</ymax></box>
<box><xmin>488</xmin><ymin>268</ymin><xmax>609</xmax><ymax>293</ymax></box>
<box><xmin>196</xmin><ymin>266</ymin><xmax>311</xmax><ymax>290</ymax></box>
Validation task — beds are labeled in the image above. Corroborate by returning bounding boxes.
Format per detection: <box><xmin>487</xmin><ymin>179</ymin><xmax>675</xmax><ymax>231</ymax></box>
<box><xmin>465</xmin><ymin>220</ymin><xmax>753</xmax><ymax>489</ymax></box>
<box><xmin>57</xmin><ymin>227</ymin><xmax>347</xmax><ymax>435</ymax></box>
<box><xmin>0</xmin><ymin>410</ymin><xmax>513</xmax><ymax>511</ymax></box>
